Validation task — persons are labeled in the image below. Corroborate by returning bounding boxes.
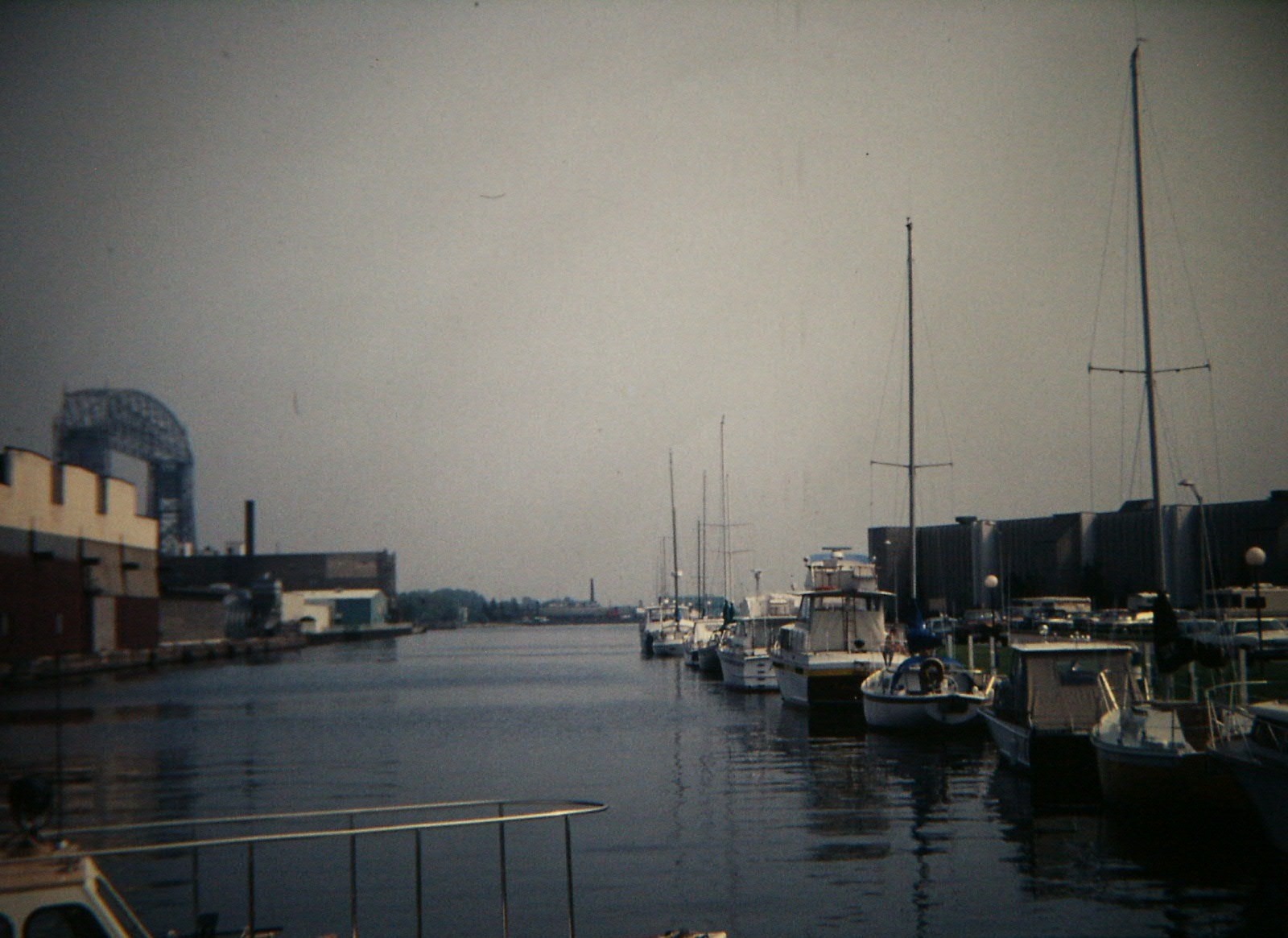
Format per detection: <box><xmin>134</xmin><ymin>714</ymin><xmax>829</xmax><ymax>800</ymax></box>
<box><xmin>883</xmin><ymin>627</ymin><xmax>897</xmax><ymax>666</ymax></box>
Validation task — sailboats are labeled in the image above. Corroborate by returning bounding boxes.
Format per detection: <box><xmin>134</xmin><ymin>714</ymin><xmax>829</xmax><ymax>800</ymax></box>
<box><xmin>1086</xmin><ymin>44</ymin><xmax>1220</xmax><ymax>819</ymax></box>
<box><xmin>859</xmin><ymin>221</ymin><xmax>996</xmax><ymax>730</ymax></box>
<box><xmin>682</xmin><ymin>415</ymin><xmax>742</xmax><ymax>672</ymax></box>
<box><xmin>652</xmin><ymin>453</ymin><xmax>695</xmax><ymax>656</ymax></box>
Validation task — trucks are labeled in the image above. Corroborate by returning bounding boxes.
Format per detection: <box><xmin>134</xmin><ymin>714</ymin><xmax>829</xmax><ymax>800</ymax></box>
<box><xmin>1204</xmin><ymin>584</ymin><xmax>1288</xmax><ymax>620</ymax></box>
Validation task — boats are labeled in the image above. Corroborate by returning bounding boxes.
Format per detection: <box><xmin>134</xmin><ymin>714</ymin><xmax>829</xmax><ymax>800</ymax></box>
<box><xmin>716</xmin><ymin>570</ymin><xmax>797</xmax><ymax>690</ymax></box>
<box><xmin>767</xmin><ymin>547</ymin><xmax>911</xmax><ymax>709</ymax></box>
<box><xmin>638</xmin><ymin>605</ymin><xmax>689</xmax><ymax>652</ymax></box>
<box><xmin>980</xmin><ymin>642</ymin><xmax>1141</xmax><ymax>778</ymax></box>
<box><xmin>1204</xmin><ymin>679</ymin><xmax>1287</xmax><ymax>854</ymax></box>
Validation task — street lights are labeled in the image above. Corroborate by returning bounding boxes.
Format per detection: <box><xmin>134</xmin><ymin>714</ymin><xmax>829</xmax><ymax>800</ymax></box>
<box><xmin>1246</xmin><ymin>548</ymin><xmax>1266</xmax><ymax>679</ymax></box>
<box><xmin>984</xmin><ymin>575</ymin><xmax>999</xmax><ymax>668</ymax></box>
<box><xmin>1178</xmin><ymin>480</ymin><xmax>1207</xmax><ymax>619</ymax></box>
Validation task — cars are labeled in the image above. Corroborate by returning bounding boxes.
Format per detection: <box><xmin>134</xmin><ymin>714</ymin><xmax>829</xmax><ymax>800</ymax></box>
<box><xmin>1199</xmin><ymin>618</ymin><xmax>1288</xmax><ymax>659</ymax></box>
<box><xmin>999</xmin><ymin>606</ymin><xmax>1217</xmax><ymax>641</ymax></box>
<box><xmin>923</xmin><ymin>616</ymin><xmax>956</xmax><ymax>642</ymax></box>
<box><xmin>955</xmin><ymin>608</ymin><xmax>1011</xmax><ymax>644</ymax></box>
